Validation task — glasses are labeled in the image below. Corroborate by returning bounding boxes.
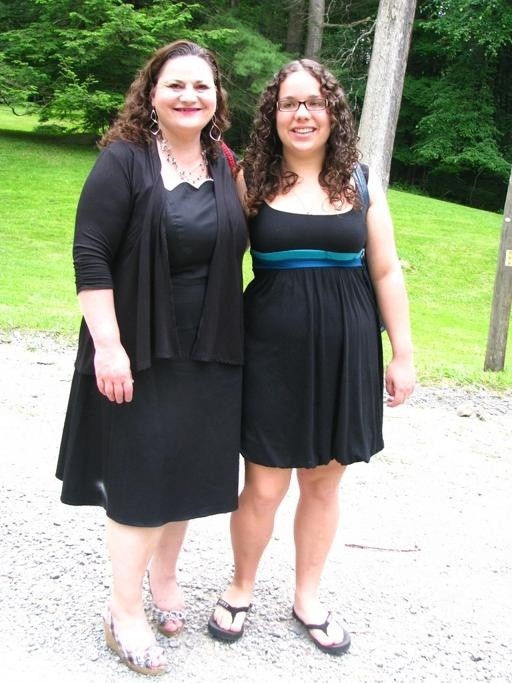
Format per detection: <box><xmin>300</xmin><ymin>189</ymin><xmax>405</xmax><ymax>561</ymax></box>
<box><xmin>276</xmin><ymin>97</ymin><xmax>329</xmax><ymax>112</ymax></box>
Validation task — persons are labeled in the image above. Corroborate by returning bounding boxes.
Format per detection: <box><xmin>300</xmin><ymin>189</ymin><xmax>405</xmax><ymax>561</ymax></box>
<box><xmin>54</xmin><ymin>38</ymin><xmax>255</xmax><ymax>676</ymax></box>
<box><xmin>206</xmin><ymin>56</ymin><xmax>418</xmax><ymax>656</ymax></box>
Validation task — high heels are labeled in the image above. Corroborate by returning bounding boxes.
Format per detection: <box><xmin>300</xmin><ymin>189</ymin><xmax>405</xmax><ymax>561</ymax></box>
<box><xmin>147</xmin><ymin>565</ymin><xmax>186</xmax><ymax>636</ymax></box>
<box><xmin>102</xmin><ymin>606</ymin><xmax>168</xmax><ymax>675</ymax></box>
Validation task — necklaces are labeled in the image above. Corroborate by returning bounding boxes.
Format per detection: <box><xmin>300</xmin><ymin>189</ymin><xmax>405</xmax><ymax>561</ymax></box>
<box><xmin>291</xmin><ymin>188</ymin><xmax>323</xmax><ymax>215</ymax></box>
<box><xmin>154</xmin><ymin>129</ymin><xmax>207</xmax><ymax>185</ymax></box>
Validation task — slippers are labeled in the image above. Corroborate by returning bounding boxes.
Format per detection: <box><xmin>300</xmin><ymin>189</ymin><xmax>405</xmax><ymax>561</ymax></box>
<box><xmin>291</xmin><ymin>599</ymin><xmax>351</xmax><ymax>654</ymax></box>
<box><xmin>207</xmin><ymin>592</ymin><xmax>252</xmax><ymax>641</ymax></box>
